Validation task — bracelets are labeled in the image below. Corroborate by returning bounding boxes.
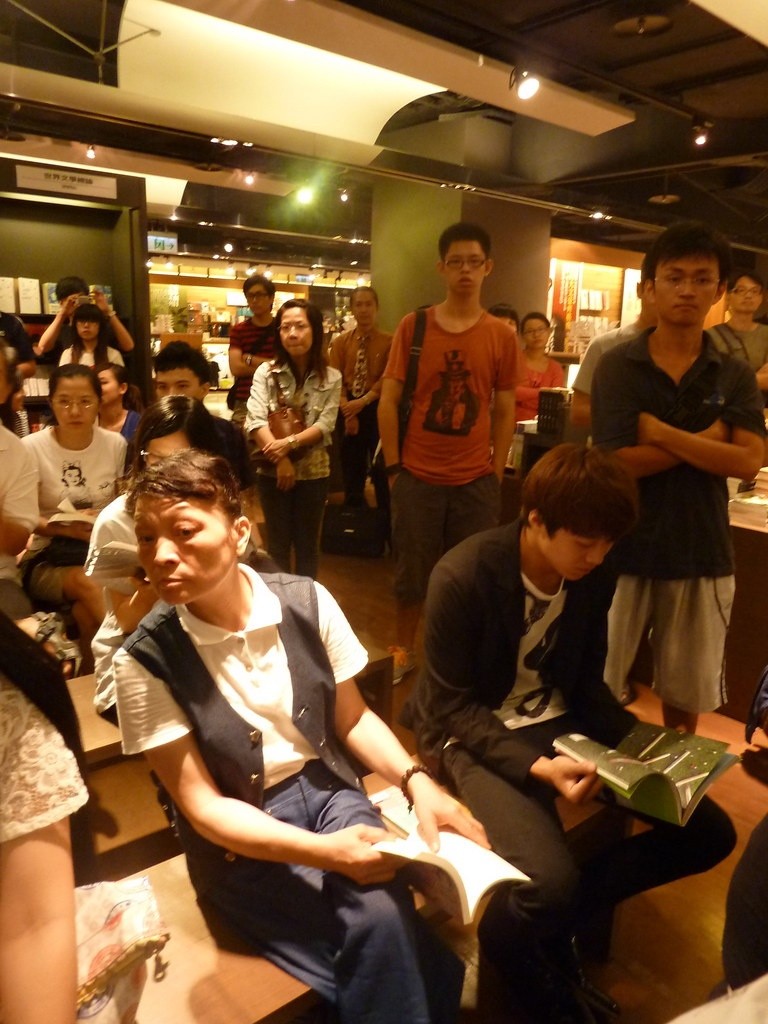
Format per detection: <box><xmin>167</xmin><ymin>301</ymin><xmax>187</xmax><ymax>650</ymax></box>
<box><xmin>402</xmin><ymin>765</ymin><xmax>435</xmax><ymax>810</ymax></box>
<box><xmin>387</xmin><ymin>463</ymin><xmax>402</xmax><ymax>477</ymax></box>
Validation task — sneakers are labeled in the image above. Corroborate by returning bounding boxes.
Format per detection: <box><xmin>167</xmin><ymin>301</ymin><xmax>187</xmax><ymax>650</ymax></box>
<box><xmin>386</xmin><ymin>646</ymin><xmax>417</xmax><ymax>685</ymax></box>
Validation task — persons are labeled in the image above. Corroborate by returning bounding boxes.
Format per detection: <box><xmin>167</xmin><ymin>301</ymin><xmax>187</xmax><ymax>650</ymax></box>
<box><xmin>0</xmin><ymin>219</ymin><xmax>768</xmax><ymax>1024</ymax></box>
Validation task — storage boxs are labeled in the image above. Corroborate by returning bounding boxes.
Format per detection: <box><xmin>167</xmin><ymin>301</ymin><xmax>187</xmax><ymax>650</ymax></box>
<box><xmin>0</xmin><ymin>277</ymin><xmax>114</xmax><ymax>316</ymax></box>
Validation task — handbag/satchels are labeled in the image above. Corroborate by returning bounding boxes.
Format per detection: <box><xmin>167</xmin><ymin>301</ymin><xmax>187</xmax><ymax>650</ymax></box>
<box><xmin>363</xmin><ymin>438</ymin><xmax>390</xmax><ymax>537</ymax></box>
<box><xmin>243</xmin><ymin>363</ymin><xmax>308</xmax><ymax>466</ymax></box>
<box><xmin>73</xmin><ymin>876</ymin><xmax>171</xmax><ymax>1024</ymax></box>
<box><xmin>227</xmin><ymin>381</ymin><xmax>239</xmax><ymax>410</ymax></box>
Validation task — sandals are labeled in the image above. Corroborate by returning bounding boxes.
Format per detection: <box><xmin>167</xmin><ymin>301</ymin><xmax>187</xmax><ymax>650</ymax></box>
<box><xmin>31</xmin><ymin>612</ymin><xmax>83</xmax><ymax>679</ymax></box>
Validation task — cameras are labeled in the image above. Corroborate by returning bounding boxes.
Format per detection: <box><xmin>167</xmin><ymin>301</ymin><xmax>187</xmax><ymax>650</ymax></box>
<box><xmin>76</xmin><ymin>296</ymin><xmax>93</xmax><ymax>306</ymax></box>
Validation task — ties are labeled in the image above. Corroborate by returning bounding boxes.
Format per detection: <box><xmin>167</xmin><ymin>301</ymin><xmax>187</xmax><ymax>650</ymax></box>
<box><xmin>352</xmin><ymin>336</ymin><xmax>369</xmax><ymax>399</ymax></box>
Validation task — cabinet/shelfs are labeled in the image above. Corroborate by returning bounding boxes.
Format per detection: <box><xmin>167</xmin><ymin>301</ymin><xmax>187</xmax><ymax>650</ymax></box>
<box><xmin>0</xmin><ymin>190</ymin><xmax>149</xmax><ymax>432</ymax></box>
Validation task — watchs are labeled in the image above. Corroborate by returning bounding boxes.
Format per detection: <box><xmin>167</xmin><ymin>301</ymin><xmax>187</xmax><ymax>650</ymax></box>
<box><xmin>105</xmin><ymin>310</ymin><xmax>117</xmax><ymax>320</ymax></box>
<box><xmin>289</xmin><ymin>433</ymin><xmax>298</xmax><ymax>450</ymax></box>
<box><xmin>246</xmin><ymin>355</ymin><xmax>254</xmax><ymax>366</ymax></box>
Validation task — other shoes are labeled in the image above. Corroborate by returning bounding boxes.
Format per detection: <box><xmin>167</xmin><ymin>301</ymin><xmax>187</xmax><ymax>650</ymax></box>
<box><xmin>509</xmin><ymin>960</ymin><xmax>620</xmax><ymax>1024</ymax></box>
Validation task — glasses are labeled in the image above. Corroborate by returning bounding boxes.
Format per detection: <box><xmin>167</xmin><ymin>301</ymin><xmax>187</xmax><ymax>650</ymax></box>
<box><xmin>654</xmin><ymin>273</ymin><xmax>720</xmax><ymax>291</ymax></box>
<box><xmin>50</xmin><ymin>397</ymin><xmax>100</xmax><ymax>409</ymax></box>
<box><xmin>730</xmin><ymin>285</ymin><xmax>763</xmax><ymax>296</ymax></box>
<box><xmin>246</xmin><ymin>292</ymin><xmax>272</xmax><ymax>299</ymax></box>
<box><xmin>522</xmin><ymin>325</ymin><xmax>550</xmax><ymax>337</ymax></box>
<box><xmin>279</xmin><ymin>322</ymin><xmax>309</xmax><ymax>333</ymax></box>
<box><xmin>140</xmin><ymin>449</ymin><xmax>165</xmax><ymax>467</ymax></box>
<box><xmin>442</xmin><ymin>256</ymin><xmax>488</xmax><ymax>268</ymax></box>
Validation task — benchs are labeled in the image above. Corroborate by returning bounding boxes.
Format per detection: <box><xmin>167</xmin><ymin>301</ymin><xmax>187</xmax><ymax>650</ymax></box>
<box><xmin>72</xmin><ymin>754</ymin><xmax>636</xmax><ymax>1024</ymax></box>
<box><xmin>64</xmin><ymin>631</ymin><xmax>394</xmax><ymax>767</ymax></box>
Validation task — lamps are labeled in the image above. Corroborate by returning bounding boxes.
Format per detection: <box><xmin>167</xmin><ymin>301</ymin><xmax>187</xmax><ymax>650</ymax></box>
<box><xmin>194</xmin><ymin>141</ymin><xmax>222</xmax><ymax>172</ymax></box>
<box><xmin>145</xmin><ymin>255</ymin><xmax>153</xmax><ymax>267</ymax></box>
<box><xmin>263</xmin><ymin>263</ymin><xmax>272</xmax><ymax>278</ymax></box>
<box><xmin>342</xmin><ymin>251</ymin><xmax>360</xmax><ymax>267</ymax></box>
<box><xmin>164</xmin><ymin>257</ymin><xmax>174</xmax><ymax>269</ymax></box>
<box><xmin>355</xmin><ymin>272</ymin><xmax>364</xmax><ymax>285</ymax></box>
<box><xmin>226</xmin><ymin>261</ymin><xmax>235</xmax><ymax>274</ymax></box>
<box><xmin>0</xmin><ymin>105</ymin><xmax>25</xmax><ymax>142</ymax></box>
<box><xmin>612</xmin><ymin>0</ymin><xmax>672</xmax><ymax>37</ymax></box>
<box><xmin>246</xmin><ymin>263</ymin><xmax>256</xmax><ymax>275</ymax></box>
<box><xmin>84</xmin><ymin>145</ymin><xmax>95</xmax><ymax>159</ymax></box>
<box><xmin>691</xmin><ymin>122</ymin><xmax>711</xmax><ymax>149</ymax></box>
<box><xmin>307</xmin><ymin>268</ymin><xmax>314</xmax><ymax>282</ymax></box>
<box><xmin>246</xmin><ymin>172</ymin><xmax>258</xmax><ymax>184</ymax></box>
<box><xmin>649</xmin><ymin>174</ymin><xmax>682</xmax><ymax>205</ymax></box>
<box><xmin>340</xmin><ymin>189</ymin><xmax>349</xmax><ymax>200</ymax></box>
<box><xmin>510</xmin><ymin>62</ymin><xmax>540</xmax><ymax>102</ymax></box>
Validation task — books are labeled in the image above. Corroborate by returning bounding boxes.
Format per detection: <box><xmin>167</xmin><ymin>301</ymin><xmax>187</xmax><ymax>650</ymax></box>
<box><xmin>553</xmin><ymin>720</ymin><xmax>744</xmax><ymax>828</ymax></box>
<box><xmin>370</xmin><ymin>824</ymin><xmax>533</xmax><ymax>926</ymax></box>
